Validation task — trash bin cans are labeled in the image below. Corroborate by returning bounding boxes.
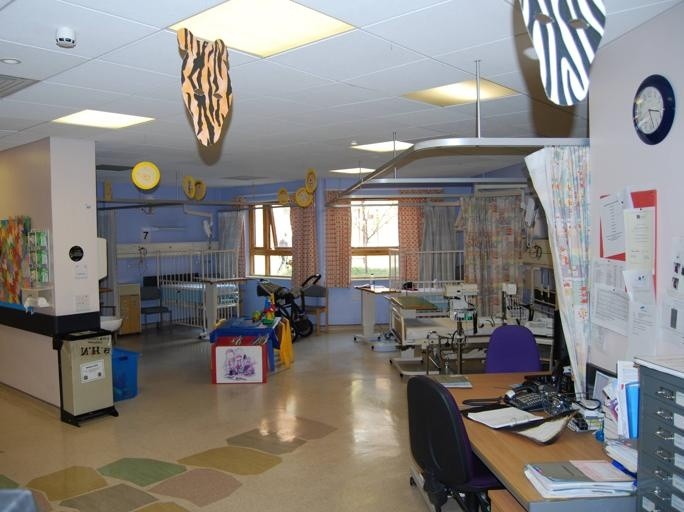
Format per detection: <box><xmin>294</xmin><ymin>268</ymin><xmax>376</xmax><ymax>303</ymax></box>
<box><xmin>54</xmin><ymin>328</ymin><xmax>119</xmax><ymax>428</ymax></box>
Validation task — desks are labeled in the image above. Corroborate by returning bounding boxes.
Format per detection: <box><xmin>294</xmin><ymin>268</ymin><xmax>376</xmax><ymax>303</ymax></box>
<box><xmin>198</xmin><ymin>277</ymin><xmax>254</xmax><ymax>341</ymax></box>
<box><xmin>209</xmin><ymin>317</ymin><xmax>283</xmax><ymax>372</ymax></box>
<box><xmin>353</xmin><ymin>285</ymin><xmax>438</xmax><ymax>363</ymax></box>
<box><xmin>428</xmin><ymin>372</ymin><xmax>638</xmax><ymax>511</ymax></box>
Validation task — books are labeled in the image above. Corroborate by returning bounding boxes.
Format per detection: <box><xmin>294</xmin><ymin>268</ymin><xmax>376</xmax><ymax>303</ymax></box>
<box><xmin>603</xmin><ymin>430</ymin><xmax>647</xmax><ymax>471</ymax></box>
<box><xmin>522</xmin><ymin>457</ymin><xmax>637</xmax><ymax>500</ymax></box>
<box><xmin>600</xmin><ymin>362</ymin><xmax>640</xmax><ymax>446</ymax></box>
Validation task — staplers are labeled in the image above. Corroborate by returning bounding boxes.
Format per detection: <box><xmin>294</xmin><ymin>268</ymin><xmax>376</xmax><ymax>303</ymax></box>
<box><xmin>569</xmin><ymin>412</ymin><xmax>588</xmax><ymax>432</ymax></box>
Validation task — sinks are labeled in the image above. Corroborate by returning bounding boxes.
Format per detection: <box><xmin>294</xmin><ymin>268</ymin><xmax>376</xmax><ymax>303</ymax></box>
<box><xmin>100</xmin><ymin>315</ymin><xmax>123</xmax><ymax>330</ymax></box>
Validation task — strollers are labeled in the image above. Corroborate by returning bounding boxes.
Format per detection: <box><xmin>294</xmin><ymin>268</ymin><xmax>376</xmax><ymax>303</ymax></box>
<box><xmin>255</xmin><ymin>272</ymin><xmax>321</xmax><ymax>344</ymax></box>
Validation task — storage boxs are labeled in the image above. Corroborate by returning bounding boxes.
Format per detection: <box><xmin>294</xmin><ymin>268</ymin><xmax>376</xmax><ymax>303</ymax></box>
<box><xmin>112</xmin><ymin>346</ymin><xmax>142</xmax><ymax>402</ymax></box>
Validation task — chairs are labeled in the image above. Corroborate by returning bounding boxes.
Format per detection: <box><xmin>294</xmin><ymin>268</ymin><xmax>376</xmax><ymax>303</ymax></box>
<box><xmin>406</xmin><ymin>374</ymin><xmax>506</xmax><ymax>512</ymax></box>
<box><xmin>140</xmin><ymin>286</ymin><xmax>172</xmax><ymax>334</ymax></box>
<box><xmin>291</xmin><ymin>285</ymin><xmax>329</xmax><ymax>337</ymax></box>
<box><xmin>485</xmin><ymin>325</ymin><xmax>542</xmax><ymax>372</ymax></box>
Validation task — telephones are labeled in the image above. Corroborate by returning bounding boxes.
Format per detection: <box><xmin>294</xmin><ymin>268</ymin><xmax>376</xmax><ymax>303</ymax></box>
<box><xmin>504</xmin><ymin>386</ymin><xmax>549</xmax><ymax>411</ymax></box>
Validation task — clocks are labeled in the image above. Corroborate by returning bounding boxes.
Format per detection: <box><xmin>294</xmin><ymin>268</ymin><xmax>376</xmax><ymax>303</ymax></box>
<box><xmin>631</xmin><ymin>74</ymin><xmax>675</xmax><ymax>145</ymax></box>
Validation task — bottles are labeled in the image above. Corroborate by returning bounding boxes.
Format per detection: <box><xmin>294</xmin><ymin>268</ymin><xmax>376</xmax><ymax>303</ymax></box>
<box><xmin>369</xmin><ymin>273</ymin><xmax>374</xmax><ymax>290</ymax></box>
